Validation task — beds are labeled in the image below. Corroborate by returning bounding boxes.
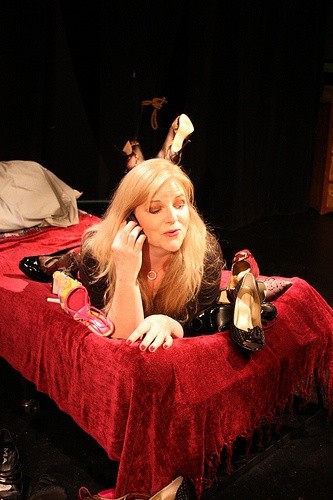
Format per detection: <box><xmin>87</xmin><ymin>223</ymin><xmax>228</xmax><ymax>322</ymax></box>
<box><xmin>1</xmin><ymin>159</ymin><xmax>333</xmax><ymax>500</ymax></box>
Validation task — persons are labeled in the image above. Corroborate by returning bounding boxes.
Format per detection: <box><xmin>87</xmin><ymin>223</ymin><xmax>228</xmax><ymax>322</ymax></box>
<box><xmin>77</xmin><ymin>112</ymin><xmax>223</xmax><ymax>353</ymax></box>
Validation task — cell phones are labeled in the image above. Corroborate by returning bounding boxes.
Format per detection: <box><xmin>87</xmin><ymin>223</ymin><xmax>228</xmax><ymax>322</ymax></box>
<box><xmin>124</xmin><ymin>211</ymin><xmax>145</xmax><ymax>244</ymax></box>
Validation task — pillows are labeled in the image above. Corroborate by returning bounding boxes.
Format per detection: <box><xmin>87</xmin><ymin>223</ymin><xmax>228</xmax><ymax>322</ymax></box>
<box><xmin>0</xmin><ymin>158</ymin><xmax>87</xmax><ymax>233</ymax></box>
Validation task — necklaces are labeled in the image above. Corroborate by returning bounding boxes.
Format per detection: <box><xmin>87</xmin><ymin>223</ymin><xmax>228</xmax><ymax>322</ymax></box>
<box><xmin>139</xmin><ymin>233</ymin><xmax>179</xmax><ymax>280</ymax></box>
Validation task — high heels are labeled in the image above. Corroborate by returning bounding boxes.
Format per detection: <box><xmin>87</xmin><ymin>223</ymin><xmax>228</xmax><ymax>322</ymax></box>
<box><xmin>217</xmin><ymin>277</ymin><xmax>293</xmax><ymax>303</ymax></box>
<box><xmin>52</xmin><ymin>270</ymin><xmax>114</xmax><ymax>337</ymax></box>
<box><xmin>38</xmin><ymin>246</ymin><xmax>81</xmax><ymax>279</ymax></box>
<box><xmin>113</xmin><ymin>134</ymin><xmax>144</xmax><ymax>171</ymax></box>
<box><xmin>18</xmin><ymin>244</ymin><xmax>83</xmax><ymax>282</ymax></box>
<box><xmin>159</xmin><ymin>113</ymin><xmax>194</xmax><ymax>164</ymax></box>
<box><xmin>191</xmin><ymin>302</ymin><xmax>277</xmax><ymax>335</ymax></box>
<box><xmin>232</xmin><ymin>273</ymin><xmax>265</xmax><ymax>350</ymax></box>
<box><xmin>232</xmin><ymin>249</ymin><xmax>258</xmax><ymax>285</ymax></box>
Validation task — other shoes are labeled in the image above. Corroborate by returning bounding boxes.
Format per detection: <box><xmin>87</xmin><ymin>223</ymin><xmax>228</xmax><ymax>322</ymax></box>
<box><xmin>0</xmin><ymin>423</ymin><xmax>197</xmax><ymax>500</ymax></box>
